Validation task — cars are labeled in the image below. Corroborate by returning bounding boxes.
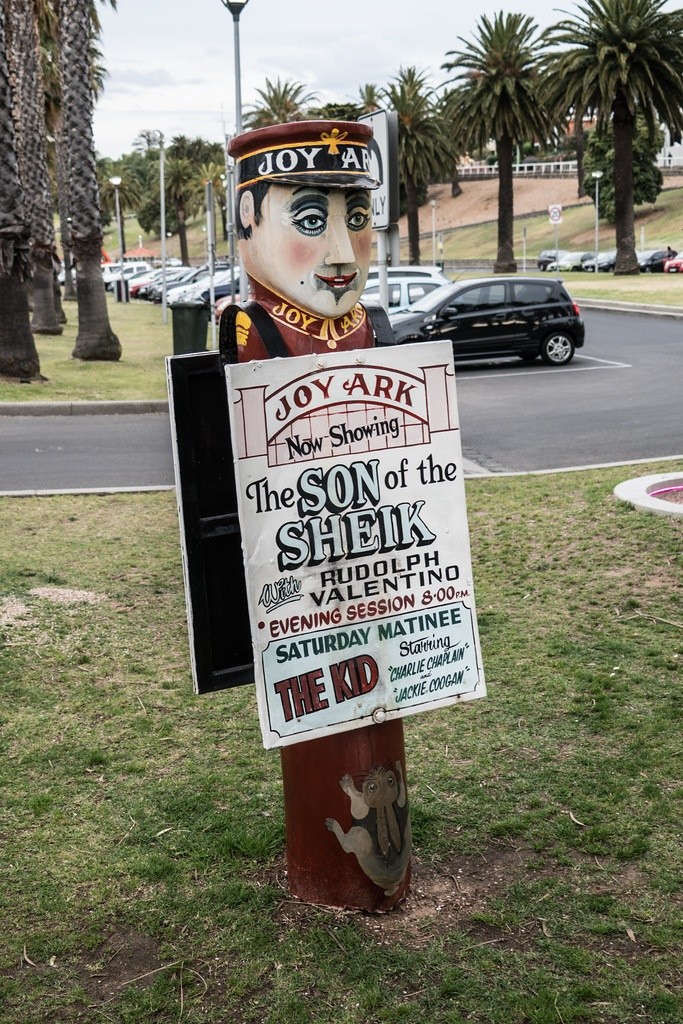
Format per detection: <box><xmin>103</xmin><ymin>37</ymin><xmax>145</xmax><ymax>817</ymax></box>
<box><xmin>373</xmin><ymin>275</ymin><xmax>586</xmax><ymax>366</ymax></box>
<box><xmin>103</xmin><ymin>262</ymin><xmax>255</xmax><ymax>324</ymax></box>
<box><xmin>664</xmin><ymin>252</ymin><xmax>683</xmax><ymax>274</ymax></box>
<box><xmin>636</xmin><ymin>250</ymin><xmax>677</xmax><ymax>273</ymax></box>
<box><xmin>581</xmin><ymin>252</ymin><xmax>618</xmax><ymax>272</ymax></box>
<box><xmin>537</xmin><ymin>250</ymin><xmax>571</xmax><ymax>270</ymax></box>
<box><xmin>366</xmin><ymin>266</ymin><xmax>451</xmax><ymax>284</ymax></box>
<box><xmin>358</xmin><ymin>277</ymin><xmax>463</xmax><ymax>315</ymax></box>
<box><xmin>548</xmin><ymin>252</ymin><xmax>586</xmax><ymax>271</ymax></box>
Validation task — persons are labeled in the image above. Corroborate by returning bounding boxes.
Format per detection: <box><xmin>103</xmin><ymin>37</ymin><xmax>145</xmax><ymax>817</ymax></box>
<box><xmin>222</xmin><ymin>122</ymin><xmax>385</xmax><ymax>362</ymax></box>
<box><xmin>665</xmin><ymin>246</ymin><xmax>674</xmax><ymax>259</ymax></box>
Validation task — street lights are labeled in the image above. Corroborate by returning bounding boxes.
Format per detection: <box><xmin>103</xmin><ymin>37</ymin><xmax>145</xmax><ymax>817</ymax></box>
<box><xmin>109</xmin><ymin>176</ymin><xmax>129</xmax><ymax>302</ymax></box>
<box><xmin>592</xmin><ymin>170</ymin><xmax>604</xmax><ymax>274</ymax></box>
<box><xmin>220</xmin><ymin>0</ymin><xmax>251</xmax><ymax>307</ymax></box>
<box><xmin>430</xmin><ymin>199</ymin><xmax>437</xmax><ymax>266</ymax></box>
